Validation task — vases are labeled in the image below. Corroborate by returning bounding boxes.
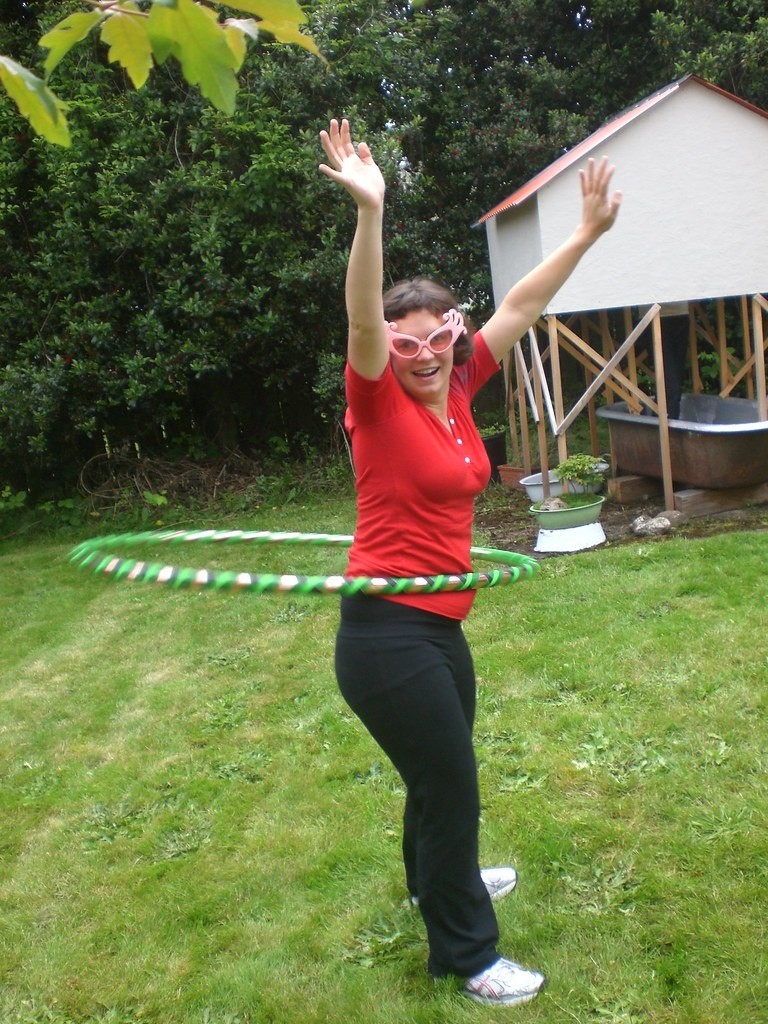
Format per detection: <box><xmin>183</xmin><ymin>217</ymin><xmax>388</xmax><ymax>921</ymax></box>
<box><xmin>519</xmin><ymin>462</ymin><xmax>609</xmax><ymax>503</ymax></box>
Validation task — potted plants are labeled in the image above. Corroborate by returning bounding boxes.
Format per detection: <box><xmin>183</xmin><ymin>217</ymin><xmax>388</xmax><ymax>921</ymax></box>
<box><xmin>475</xmin><ymin>422</ymin><xmax>508</xmax><ymax>479</ymax></box>
<box><xmin>495</xmin><ymin>427</ymin><xmax>553</xmax><ymax>491</ymax></box>
<box><xmin>530</xmin><ymin>452</ymin><xmax>607</xmax><ymax>530</ymax></box>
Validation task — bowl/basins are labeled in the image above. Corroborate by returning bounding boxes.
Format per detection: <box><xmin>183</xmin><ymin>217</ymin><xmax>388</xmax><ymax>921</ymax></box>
<box><xmin>519</xmin><ymin>463</ymin><xmax>610</xmax><ymax>502</ymax></box>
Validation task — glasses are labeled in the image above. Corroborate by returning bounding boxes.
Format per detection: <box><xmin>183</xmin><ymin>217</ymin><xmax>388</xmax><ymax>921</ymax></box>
<box><xmin>385</xmin><ymin>307</ymin><xmax>467</xmax><ymax>359</ymax></box>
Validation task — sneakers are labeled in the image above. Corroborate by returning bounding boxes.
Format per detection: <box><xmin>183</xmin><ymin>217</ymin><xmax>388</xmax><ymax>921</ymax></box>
<box><xmin>433</xmin><ymin>957</ymin><xmax>544</xmax><ymax>1009</ymax></box>
<box><xmin>411</xmin><ymin>868</ymin><xmax>517</xmax><ymax>907</ymax></box>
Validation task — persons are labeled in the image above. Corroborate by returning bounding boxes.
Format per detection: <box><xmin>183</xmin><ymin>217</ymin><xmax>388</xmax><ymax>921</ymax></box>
<box><xmin>638</xmin><ymin>300</ymin><xmax>690</xmax><ymax>420</ymax></box>
<box><xmin>319</xmin><ymin>119</ymin><xmax>622</xmax><ymax>1010</ymax></box>
